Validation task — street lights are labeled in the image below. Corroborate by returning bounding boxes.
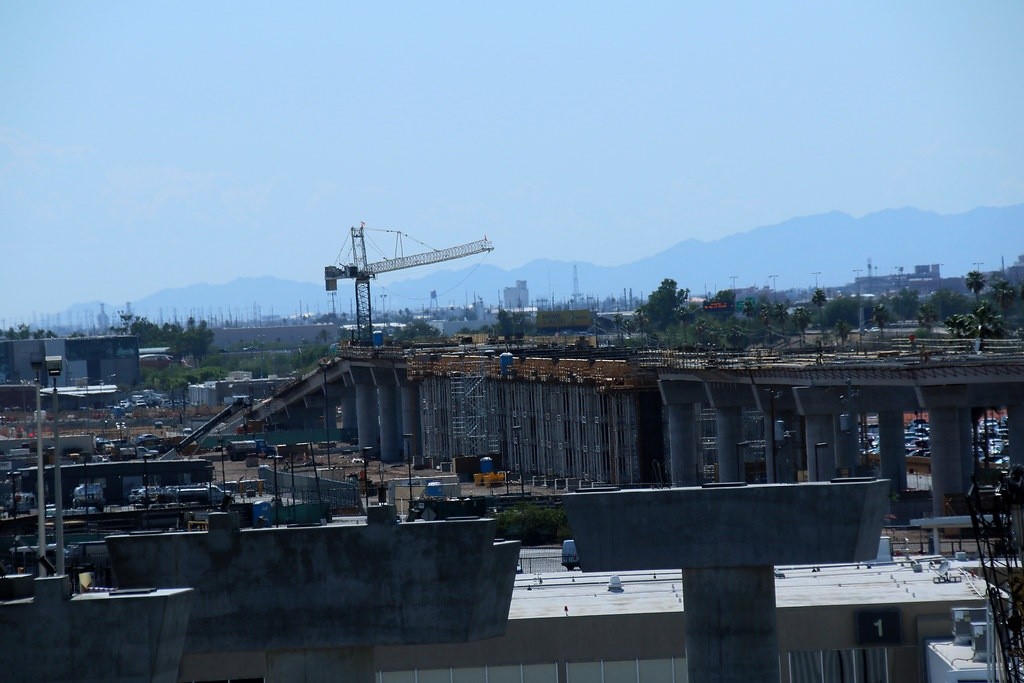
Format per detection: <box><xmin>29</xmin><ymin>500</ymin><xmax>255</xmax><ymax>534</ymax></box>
<box><xmin>319</xmin><ymin>362</ymin><xmax>337</xmax><ymax>466</ymax></box>
<box><xmin>769</xmin><ymin>275</ymin><xmax>779</xmax><ymax>304</ymax></box>
<box><xmin>811</xmin><ymin>272</ymin><xmax>821</xmax><ymax>288</ymax></box>
<box><xmin>143</xmin><ymin>453</ymin><xmax>151</xmax><ymax>509</ymax></box>
<box><xmin>6</xmin><ymin>469</ymin><xmax>23</xmax><ymax>576</ymax></box>
<box><xmin>853</xmin><ymin>269</ymin><xmax>863</xmax><ymax>296</ymax></box>
<box><xmin>363</xmin><ymin>446</ymin><xmax>373</xmax><ymax>507</ymax></box>
<box><xmin>266</xmin><ymin>455</ymin><xmax>284</xmax><ymax>528</ymax></box>
<box><xmin>729</xmin><ymin>276</ymin><xmax>739</xmax><ymax>295</ymax></box>
<box><xmin>403</xmin><ymin>433</ymin><xmax>414</xmax><ymax>502</ymax></box>
<box><xmin>511</xmin><ymin>426</ymin><xmax>527</xmax><ymax>496</ymax></box>
<box><xmin>215</xmin><ymin>442</ymin><xmax>227</xmax><ymax>498</ymax></box>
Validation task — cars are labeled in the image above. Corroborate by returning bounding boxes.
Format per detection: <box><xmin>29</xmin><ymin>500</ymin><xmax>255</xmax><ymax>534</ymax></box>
<box><xmin>861</xmin><ymin>423</ymin><xmax>880</xmax><ymax>453</ymax></box>
<box><xmin>905</xmin><ymin>421</ymin><xmax>932</xmax><ymax>458</ymax></box>
<box><xmin>979</xmin><ymin>415</ymin><xmax>1010</xmax><ymax>464</ymax></box>
<box><xmin>105</xmin><ymin>394</ymin><xmax>148</xmax><ymax>411</ymax></box>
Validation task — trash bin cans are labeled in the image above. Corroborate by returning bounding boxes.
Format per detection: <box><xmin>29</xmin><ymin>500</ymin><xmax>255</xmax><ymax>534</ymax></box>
<box><xmin>479</xmin><ymin>456</ymin><xmax>494</xmax><ymax>474</ymax></box>
<box><xmin>473</xmin><ymin>471</ymin><xmax>505</xmax><ymax>487</ymax></box>
<box><xmin>252</xmin><ymin>500</ymin><xmax>272</xmax><ymax>528</ymax></box>
<box><xmin>499</xmin><ymin>352</ymin><xmax>514</xmax><ymax>377</ymax></box>
<box><xmin>113</xmin><ymin>405</ymin><xmax>121</xmax><ymax>418</ymax></box>
<box><xmin>426</xmin><ymin>481</ymin><xmax>442</xmax><ymax>496</ymax></box>
<box><xmin>372</xmin><ymin>330</ymin><xmax>383</xmax><ymax>348</ymax></box>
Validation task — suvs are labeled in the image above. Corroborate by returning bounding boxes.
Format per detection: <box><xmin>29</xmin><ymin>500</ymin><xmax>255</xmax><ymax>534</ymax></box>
<box><xmin>129</xmin><ymin>486</ymin><xmax>164</xmax><ymax>504</ymax></box>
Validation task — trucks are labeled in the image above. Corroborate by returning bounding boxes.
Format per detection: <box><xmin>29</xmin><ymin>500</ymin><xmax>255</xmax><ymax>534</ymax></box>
<box><xmin>72</xmin><ymin>484</ymin><xmax>105</xmax><ymax>510</ymax></box>
<box><xmin>168</xmin><ymin>484</ymin><xmax>233</xmax><ymax>504</ymax></box>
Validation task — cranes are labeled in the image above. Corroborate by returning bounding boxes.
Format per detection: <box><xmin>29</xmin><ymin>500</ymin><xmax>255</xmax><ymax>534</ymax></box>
<box><xmin>322</xmin><ymin>223</ymin><xmax>499</xmax><ymax>348</ymax></box>
